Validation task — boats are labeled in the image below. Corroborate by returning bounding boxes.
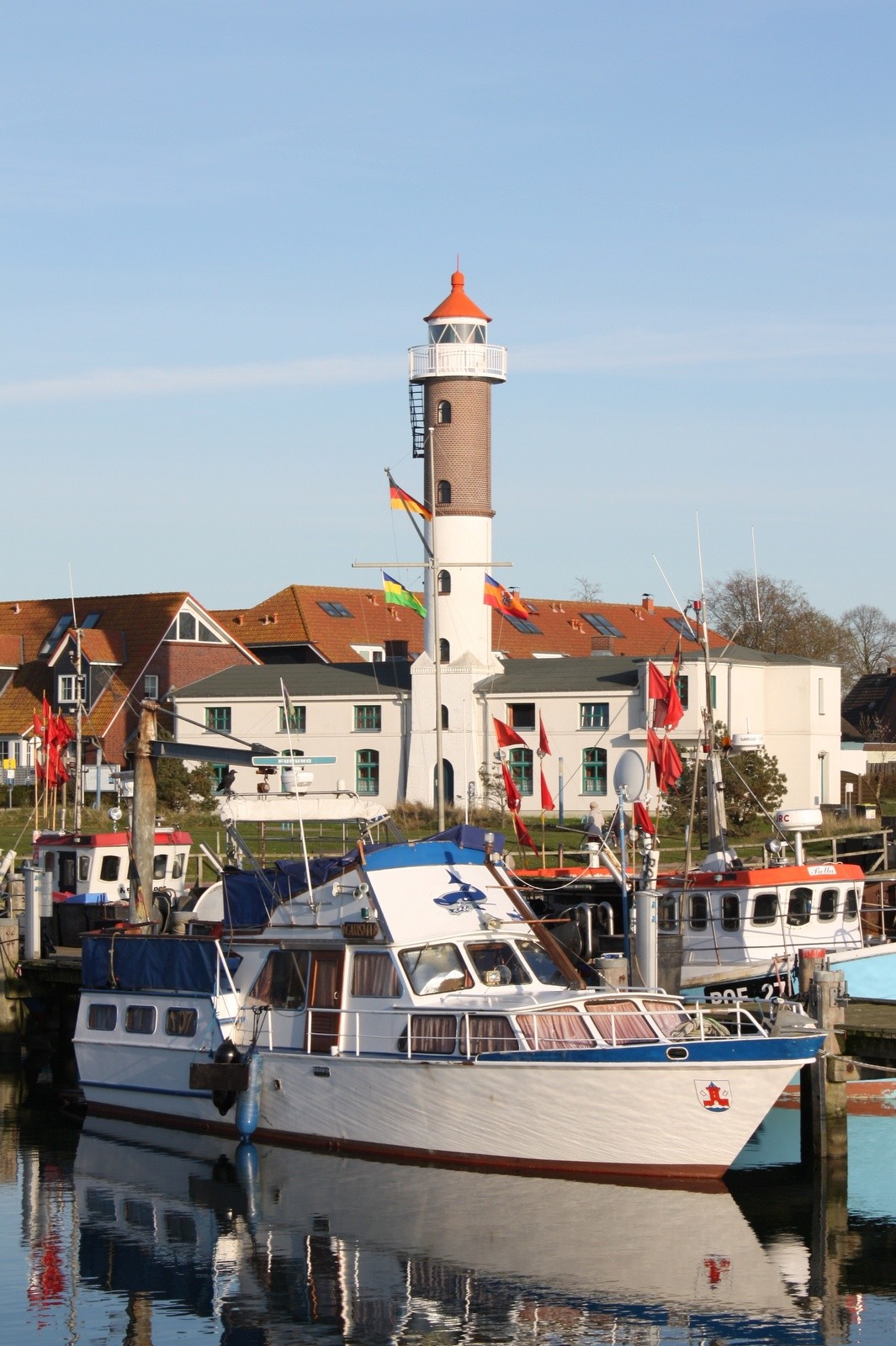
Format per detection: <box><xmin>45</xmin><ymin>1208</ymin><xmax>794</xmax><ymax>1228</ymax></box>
<box><xmin>31</xmin><ymin>827</ymin><xmax>194</xmax><ymax>906</ymax></box>
<box><xmin>70</xmin><ymin>824</ymin><xmax>828</xmax><ymax>1179</ymax></box>
<box><xmin>506</xmin><ymin>591</ymin><xmax>895</xmax><ymax>1045</ymax></box>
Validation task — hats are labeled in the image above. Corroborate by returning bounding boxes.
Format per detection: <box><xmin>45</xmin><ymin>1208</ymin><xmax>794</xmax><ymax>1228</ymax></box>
<box><xmin>589</xmin><ymin>802</ymin><xmax>599</xmax><ymax>808</ymax></box>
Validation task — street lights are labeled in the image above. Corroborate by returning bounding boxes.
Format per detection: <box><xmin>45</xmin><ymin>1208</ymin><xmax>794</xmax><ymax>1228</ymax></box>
<box><xmin>559</xmin><ymin>757</ymin><xmax>564</xmax><ymax>823</ymax></box>
<box><xmin>96</xmin><ymin>749</ymin><xmax>102</xmax><ymax>808</ymax></box>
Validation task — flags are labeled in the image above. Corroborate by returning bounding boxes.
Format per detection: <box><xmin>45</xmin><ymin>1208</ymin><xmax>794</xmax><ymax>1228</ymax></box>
<box><xmin>33</xmin><ymin>699</ymin><xmax>75</xmax><ymax>790</ymax></box>
<box><xmin>634</xmin><ymin>645</ymin><xmax>681</xmax><ymax>832</ymax></box>
<box><xmin>494</xmin><ymin>716</ymin><xmax>554</xmax><ymax>858</ymax></box>
<box><xmin>483</xmin><ymin>573</ymin><xmax>528</xmax><ymax>621</ymax></box>
<box><xmin>389</xmin><ymin>478</ymin><xmax>432</xmax><ymax>521</ymax></box>
<box><xmin>382</xmin><ymin>571</ymin><xmax>427</xmax><ymax>618</ymax></box>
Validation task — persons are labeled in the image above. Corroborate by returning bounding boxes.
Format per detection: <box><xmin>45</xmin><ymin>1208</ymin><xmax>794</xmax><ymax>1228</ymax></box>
<box><xmin>609</xmin><ymin>804</ymin><xmax>629</xmax><ymax>836</ymax></box>
<box><xmin>584</xmin><ymin>802</ymin><xmax>604</xmax><ymax>842</ymax></box>
